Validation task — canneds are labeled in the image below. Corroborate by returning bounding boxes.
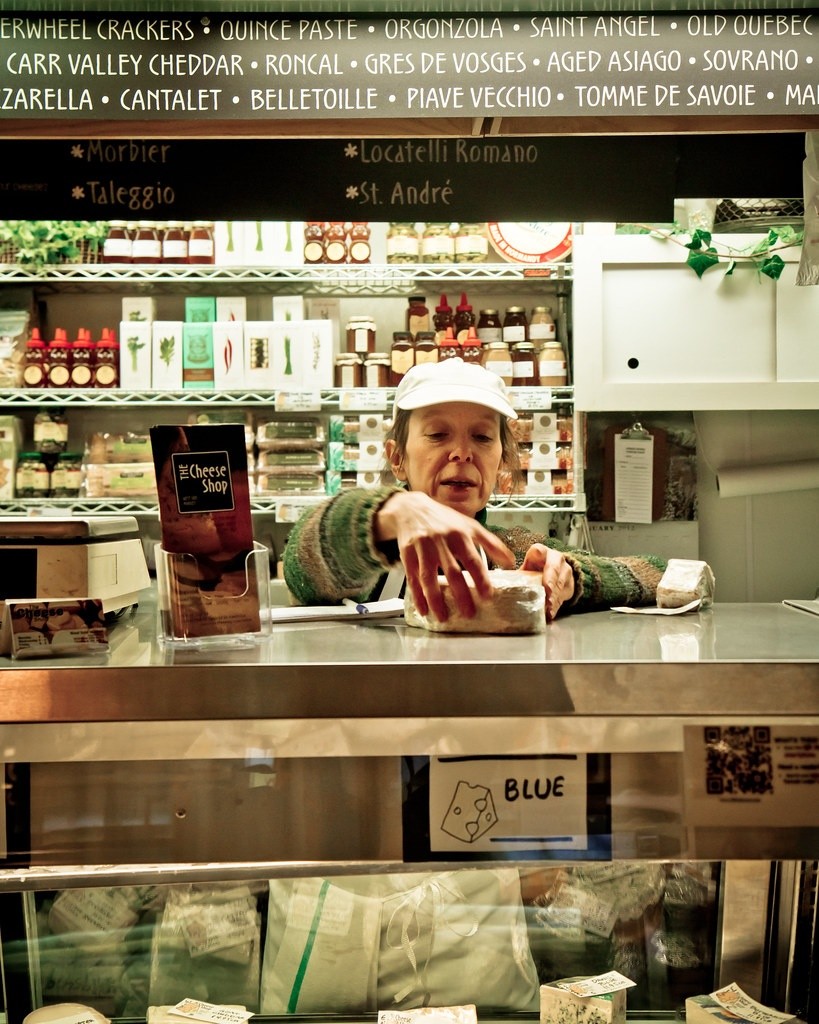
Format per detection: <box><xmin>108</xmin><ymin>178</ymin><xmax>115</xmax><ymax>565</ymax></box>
<box><xmin>14</xmin><ymin>406</ymin><xmax>81</xmax><ymax>498</ymax></box>
<box><xmin>101</xmin><ymin>221</ymin><xmax>215</xmax><ymax>266</ymax></box>
<box><xmin>477</xmin><ymin>305</ymin><xmax>567</xmax><ymax>388</ymax></box>
<box><xmin>333</xmin><ymin>295</ymin><xmax>437</xmax><ymax>388</ymax></box>
<box><xmin>302</xmin><ymin>220</ymin><xmax>492</xmax><ymax>265</ymax></box>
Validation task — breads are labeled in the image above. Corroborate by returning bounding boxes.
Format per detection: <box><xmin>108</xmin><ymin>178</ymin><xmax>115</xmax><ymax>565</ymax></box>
<box><xmin>24</xmin><ymin>976</ymin><xmax>770</xmax><ymax>1024</ymax></box>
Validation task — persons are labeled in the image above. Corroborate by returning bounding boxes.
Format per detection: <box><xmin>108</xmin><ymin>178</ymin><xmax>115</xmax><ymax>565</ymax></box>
<box><xmin>261</xmin><ymin>357</ymin><xmax>673</xmax><ymax>1016</ymax></box>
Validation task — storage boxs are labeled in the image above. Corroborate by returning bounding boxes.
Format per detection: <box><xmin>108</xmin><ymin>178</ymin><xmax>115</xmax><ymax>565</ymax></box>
<box><xmin>118</xmin><ymin>293</ymin><xmax>339</xmax><ymax>388</ymax></box>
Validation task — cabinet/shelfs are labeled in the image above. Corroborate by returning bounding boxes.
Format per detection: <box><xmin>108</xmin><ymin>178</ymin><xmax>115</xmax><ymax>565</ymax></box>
<box><xmin>571</xmin><ymin>234</ymin><xmax>819</xmax><ymax>412</ymax></box>
<box><xmin>0</xmin><ymin>260</ymin><xmax>589</xmax><ymax>518</ymax></box>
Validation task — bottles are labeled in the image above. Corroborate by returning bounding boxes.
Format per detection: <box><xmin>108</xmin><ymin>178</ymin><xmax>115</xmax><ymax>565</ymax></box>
<box><xmin>334</xmin><ymin>292</ymin><xmax>567</xmax><ymax>386</ymax></box>
<box><xmin>23</xmin><ymin>328</ymin><xmax>119</xmax><ymax>388</ymax></box>
<box><xmin>102</xmin><ymin>221</ymin><xmax>216</xmax><ymax>264</ymax></box>
<box><xmin>15</xmin><ymin>406</ymin><xmax>84</xmax><ymax>499</ymax></box>
<box><xmin>304</xmin><ymin>223</ymin><xmax>370</xmax><ymax>263</ymax></box>
<box><xmin>386</xmin><ymin>221</ymin><xmax>489</xmax><ymax>261</ymax></box>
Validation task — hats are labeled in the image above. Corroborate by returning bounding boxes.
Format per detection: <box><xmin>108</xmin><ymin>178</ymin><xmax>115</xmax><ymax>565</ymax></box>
<box><xmin>392</xmin><ymin>356</ymin><xmax>519</xmax><ymax>427</ymax></box>
